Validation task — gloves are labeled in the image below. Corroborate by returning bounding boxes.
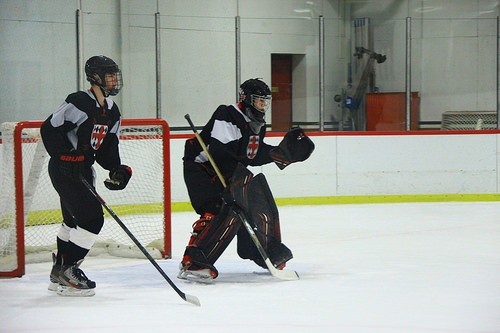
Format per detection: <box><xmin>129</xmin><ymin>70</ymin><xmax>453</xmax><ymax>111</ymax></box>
<box><xmin>66</xmin><ymin>149</ymin><xmax>93</xmax><ymax>180</ymax></box>
<box><xmin>103</xmin><ymin>163</ymin><xmax>132</xmax><ymax>190</ymax></box>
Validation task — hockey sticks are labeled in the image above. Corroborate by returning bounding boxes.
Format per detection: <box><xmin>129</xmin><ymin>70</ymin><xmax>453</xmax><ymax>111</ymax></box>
<box><xmin>78</xmin><ymin>172</ymin><xmax>202</xmax><ymax>307</ymax></box>
<box><xmin>184</xmin><ymin>114</ymin><xmax>302</xmax><ymax>281</ymax></box>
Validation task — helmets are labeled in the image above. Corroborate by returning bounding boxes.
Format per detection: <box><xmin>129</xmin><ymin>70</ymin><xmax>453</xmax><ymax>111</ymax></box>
<box><xmin>239</xmin><ymin>79</ymin><xmax>273</xmax><ymax>124</ymax></box>
<box><xmin>85</xmin><ymin>55</ymin><xmax>124</xmax><ymax>96</ymax></box>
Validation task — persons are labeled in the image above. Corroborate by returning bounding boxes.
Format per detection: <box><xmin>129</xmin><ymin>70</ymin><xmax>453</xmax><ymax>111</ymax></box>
<box><xmin>40</xmin><ymin>55</ymin><xmax>133</xmax><ymax>290</ymax></box>
<box><xmin>180</xmin><ymin>78</ymin><xmax>314</xmax><ymax>279</ymax></box>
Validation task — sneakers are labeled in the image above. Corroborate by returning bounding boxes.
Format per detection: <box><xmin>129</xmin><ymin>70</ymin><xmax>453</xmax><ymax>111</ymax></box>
<box><xmin>177</xmin><ymin>262</ymin><xmax>213</xmax><ymax>283</ymax></box>
<box><xmin>47</xmin><ymin>252</ymin><xmax>96</xmax><ymax>297</ymax></box>
